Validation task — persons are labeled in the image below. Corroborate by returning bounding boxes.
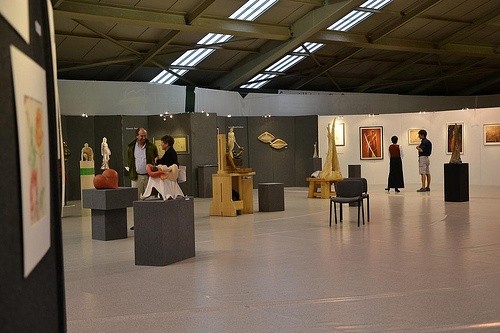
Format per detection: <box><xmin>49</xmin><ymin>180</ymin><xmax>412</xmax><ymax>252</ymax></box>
<box><xmin>154</xmin><ymin>135</ymin><xmax>179</xmax><ymax>169</ymax></box>
<box><xmin>128</xmin><ymin>127</ymin><xmax>159</xmax><ymax>230</ymax></box>
<box><xmin>227</xmin><ymin>127</ymin><xmax>235</xmax><ymax>159</ymax></box>
<box><xmin>81</xmin><ymin>142</ymin><xmax>94</xmax><ymax>161</ymax></box>
<box><xmin>101</xmin><ymin>137</ymin><xmax>111</xmax><ymax>170</ymax></box>
<box><xmin>416</xmin><ymin>130</ymin><xmax>432</xmax><ymax>192</ymax></box>
<box><xmin>385</xmin><ymin>135</ymin><xmax>405</xmax><ymax>192</ymax></box>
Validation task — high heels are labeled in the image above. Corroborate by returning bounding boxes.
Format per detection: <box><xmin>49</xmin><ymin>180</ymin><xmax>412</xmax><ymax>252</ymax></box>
<box><xmin>395</xmin><ymin>188</ymin><xmax>400</xmax><ymax>192</ymax></box>
<box><xmin>385</xmin><ymin>188</ymin><xmax>390</xmax><ymax>192</ymax></box>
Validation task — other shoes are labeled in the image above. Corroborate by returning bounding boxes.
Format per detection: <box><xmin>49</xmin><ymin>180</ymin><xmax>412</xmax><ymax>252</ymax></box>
<box><xmin>130</xmin><ymin>225</ymin><xmax>134</xmax><ymax>230</ymax></box>
<box><xmin>417</xmin><ymin>188</ymin><xmax>426</xmax><ymax>192</ymax></box>
<box><xmin>425</xmin><ymin>186</ymin><xmax>430</xmax><ymax>191</ymax></box>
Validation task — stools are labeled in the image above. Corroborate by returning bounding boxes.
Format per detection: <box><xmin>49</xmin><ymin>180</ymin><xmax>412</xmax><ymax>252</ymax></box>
<box><xmin>258</xmin><ymin>183</ymin><xmax>285</xmax><ymax>212</ymax></box>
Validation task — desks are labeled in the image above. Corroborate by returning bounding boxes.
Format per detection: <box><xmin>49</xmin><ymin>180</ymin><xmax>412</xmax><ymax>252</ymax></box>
<box><xmin>197</xmin><ymin>165</ymin><xmax>218</xmax><ymax>198</ymax></box>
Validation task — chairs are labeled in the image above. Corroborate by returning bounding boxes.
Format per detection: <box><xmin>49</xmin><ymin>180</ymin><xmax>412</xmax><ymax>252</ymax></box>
<box><xmin>329</xmin><ymin>178</ymin><xmax>370</xmax><ymax>227</ymax></box>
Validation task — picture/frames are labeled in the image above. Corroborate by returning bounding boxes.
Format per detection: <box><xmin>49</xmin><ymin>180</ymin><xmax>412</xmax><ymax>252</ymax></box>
<box><xmin>445</xmin><ymin>122</ymin><xmax>464</xmax><ymax>155</ymax></box>
<box><xmin>483</xmin><ymin>123</ymin><xmax>500</xmax><ymax>146</ymax></box>
<box><xmin>408</xmin><ymin>128</ymin><xmax>422</xmax><ymax>145</ymax></box>
<box><xmin>328</xmin><ymin>123</ymin><xmax>345</xmax><ymax>146</ymax></box>
<box><xmin>170</xmin><ymin>135</ymin><xmax>190</xmax><ymax>154</ymax></box>
<box><xmin>359</xmin><ymin>125</ymin><xmax>384</xmax><ymax>160</ymax></box>
